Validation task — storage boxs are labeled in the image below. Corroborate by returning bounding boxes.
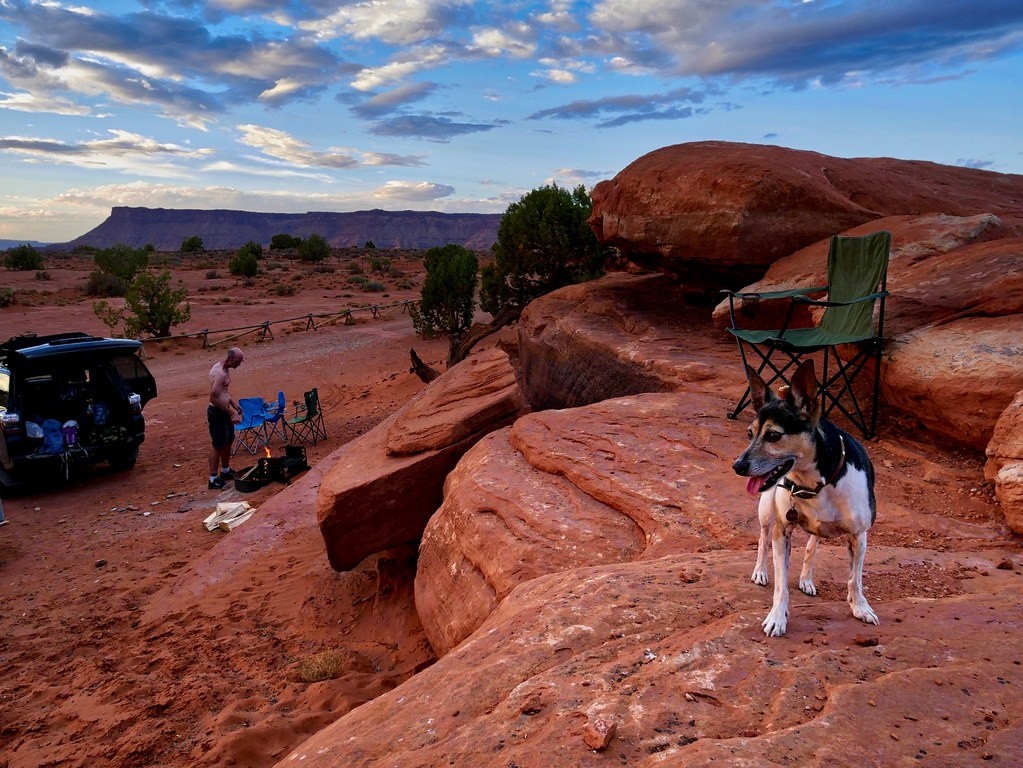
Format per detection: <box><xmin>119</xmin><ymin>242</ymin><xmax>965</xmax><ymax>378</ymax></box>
<box><xmin>277</xmin><ymin>456</ymin><xmax>308</xmax><ymax>483</ymax></box>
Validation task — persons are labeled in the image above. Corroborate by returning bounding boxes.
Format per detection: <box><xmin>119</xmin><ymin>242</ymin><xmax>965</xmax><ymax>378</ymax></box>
<box><xmin>207</xmin><ymin>347</ymin><xmax>243</xmax><ymax>488</ymax></box>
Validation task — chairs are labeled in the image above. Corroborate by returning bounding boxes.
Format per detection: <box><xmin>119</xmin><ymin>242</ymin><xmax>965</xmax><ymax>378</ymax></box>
<box><xmin>233</xmin><ymin>398</ymin><xmax>270</xmax><ymax>456</ymax></box>
<box><xmin>264</xmin><ymin>391</ymin><xmax>288</xmax><ymax>443</ymax></box>
<box><xmin>717</xmin><ymin>231</ymin><xmax>892</xmax><ymax>441</ymax></box>
<box><xmin>281</xmin><ymin>387</ymin><xmax>328</xmax><ymax>448</ymax></box>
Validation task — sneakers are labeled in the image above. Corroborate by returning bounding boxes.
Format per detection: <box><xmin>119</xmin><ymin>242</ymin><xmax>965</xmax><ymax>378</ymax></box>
<box><xmin>220</xmin><ymin>468</ymin><xmax>237</xmax><ymax>480</ymax></box>
<box><xmin>208</xmin><ymin>476</ymin><xmax>226</xmax><ymax>489</ymax></box>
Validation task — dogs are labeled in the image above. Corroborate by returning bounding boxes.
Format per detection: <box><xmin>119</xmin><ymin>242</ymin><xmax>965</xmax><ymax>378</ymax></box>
<box><xmin>731</xmin><ymin>358</ymin><xmax>880</xmax><ymax>639</ymax></box>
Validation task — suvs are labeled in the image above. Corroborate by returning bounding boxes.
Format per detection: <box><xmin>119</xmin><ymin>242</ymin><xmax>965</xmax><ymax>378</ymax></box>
<box><xmin>0</xmin><ymin>331</ymin><xmax>159</xmax><ymax>482</ymax></box>
